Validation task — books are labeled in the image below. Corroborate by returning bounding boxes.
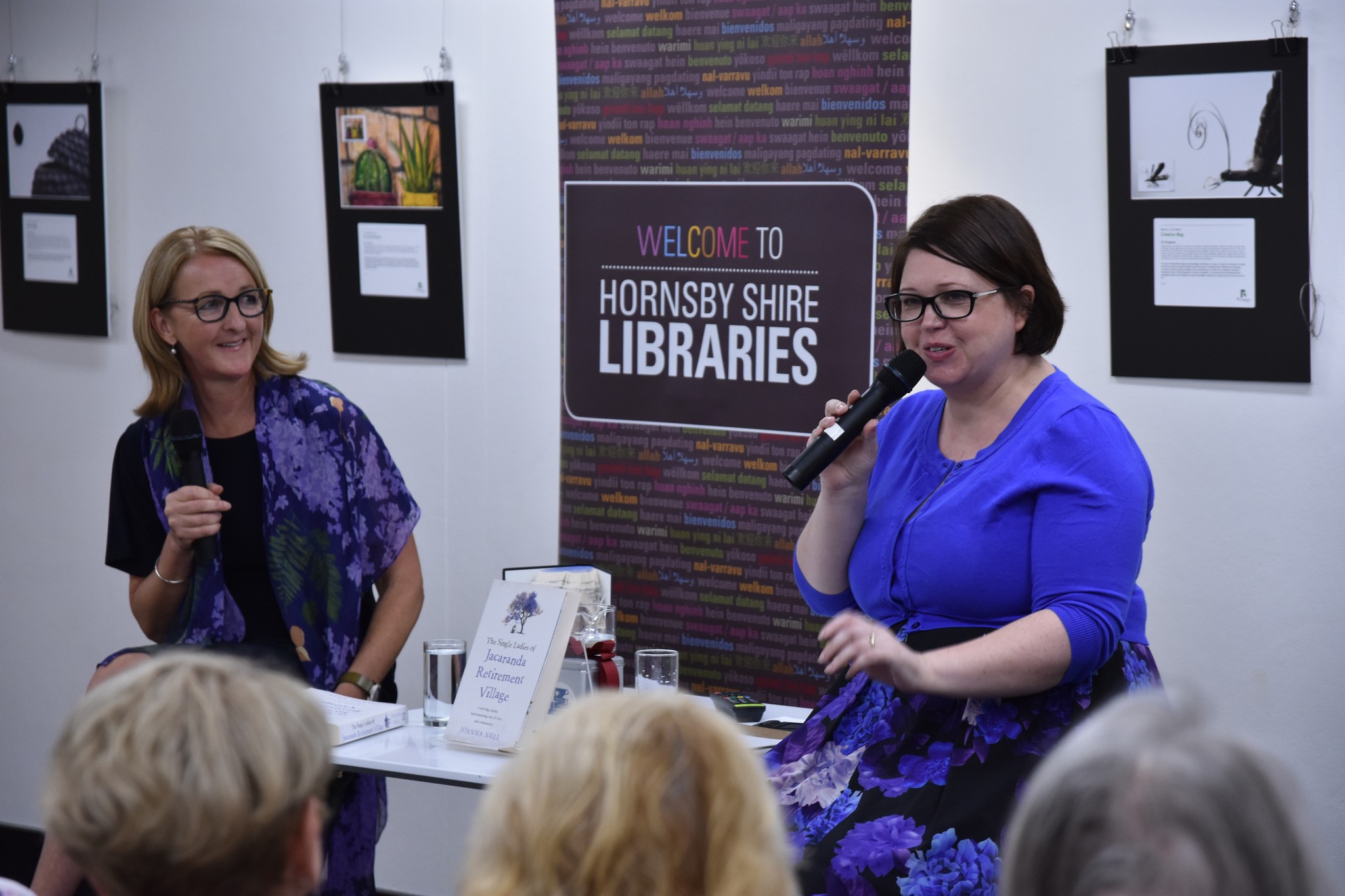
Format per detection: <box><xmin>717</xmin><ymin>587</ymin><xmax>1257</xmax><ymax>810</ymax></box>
<box><xmin>437</xmin><ymin>579</ymin><xmax>581</xmax><ymax>759</ymax></box>
<box><xmin>304</xmin><ymin>687</ymin><xmax>408</xmax><ymax>747</ymax></box>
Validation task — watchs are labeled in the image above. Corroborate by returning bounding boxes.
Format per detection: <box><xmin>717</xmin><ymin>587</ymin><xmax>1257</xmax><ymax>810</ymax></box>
<box><xmin>337</xmin><ymin>671</ymin><xmax>382</xmax><ymax>702</ymax></box>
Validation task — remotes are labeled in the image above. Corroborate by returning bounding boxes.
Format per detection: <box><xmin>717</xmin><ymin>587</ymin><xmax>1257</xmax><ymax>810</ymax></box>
<box><xmin>709</xmin><ymin>690</ymin><xmax>765</xmax><ymax>722</ymax></box>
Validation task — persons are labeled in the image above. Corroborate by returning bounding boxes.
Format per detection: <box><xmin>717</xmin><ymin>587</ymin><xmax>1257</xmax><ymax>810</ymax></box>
<box><xmin>29</xmin><ymin>225</ymin><xmax>425</xmax><ymax>896</ymax></box>
<box><xmin>467</xmin><ymin>683</ymin><xmax>801</xmax><ymax>896</ymax></box>
<box><xmin>40</xmin><ymin>646</ymin><xmax>334</xmax><ymax>896</ymax></box>
<box><xmin>762</xmin><ymin>195</ymin><xmax>1162</xmax><ymax>896</ymax></box>
<box><xmin>994</xmin><ymin>675</ymin><xmax>1332</xmax><ymax>896</ymax></box>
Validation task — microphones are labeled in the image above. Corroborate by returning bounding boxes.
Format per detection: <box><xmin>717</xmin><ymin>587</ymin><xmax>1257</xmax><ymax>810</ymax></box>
<box><xmin>170</xmin><ymin>411</ymin><xmax>220</xmax><ymax>564</ymax></box>
<box><xmin>784</xmin><ymin>350</ymin><xmax>928</xmax><ymax>492</ymax></box>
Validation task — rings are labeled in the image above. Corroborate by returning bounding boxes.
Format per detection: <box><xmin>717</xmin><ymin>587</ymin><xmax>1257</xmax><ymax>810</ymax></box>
<box><xmin>869</xmin><ymin>632</ymin><xmax>875</xmax><ymax>649</ymax></box>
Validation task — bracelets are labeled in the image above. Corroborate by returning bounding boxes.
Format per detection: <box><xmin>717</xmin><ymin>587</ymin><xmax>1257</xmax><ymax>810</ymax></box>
<box><xmin>154</xmin><ymin>555</ymin><xmax>188</xmax><ymax>584</ymax></box>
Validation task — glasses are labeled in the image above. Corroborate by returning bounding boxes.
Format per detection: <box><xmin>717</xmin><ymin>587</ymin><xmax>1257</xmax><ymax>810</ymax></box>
<box><xmin>156</xmin><ymin>289</ymin><xmax>273</xmax><ymax>323</ymax></box>
<box><xmin>884</xmin><ymin>285</ymin><xmax>1024</xmax><ymax>323</ymax></box>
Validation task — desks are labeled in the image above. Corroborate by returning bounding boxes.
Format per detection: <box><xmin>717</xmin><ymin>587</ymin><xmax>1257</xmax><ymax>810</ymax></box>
<box><xmin>325</xmin><ymin>693</ymin><xmax>815</xmax><ymax>797</ymax></box>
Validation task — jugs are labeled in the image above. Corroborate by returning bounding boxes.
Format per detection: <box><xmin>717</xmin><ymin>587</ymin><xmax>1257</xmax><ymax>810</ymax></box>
<box><xmin>564</xmin><ymin>603</ymin><xmax>617</xmax><ymax>656</ymax></box>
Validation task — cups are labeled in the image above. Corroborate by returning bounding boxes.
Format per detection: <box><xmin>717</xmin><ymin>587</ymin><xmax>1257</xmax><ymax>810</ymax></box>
<box><xmin>634</xmin><ymin>649</ymin><xmax>679</xmax><ymax>692</ymax></box>
<box><xmin>423</xmin><ymin>640</ymin><xmax>467</xmax><ymax>726</ymax></box>
